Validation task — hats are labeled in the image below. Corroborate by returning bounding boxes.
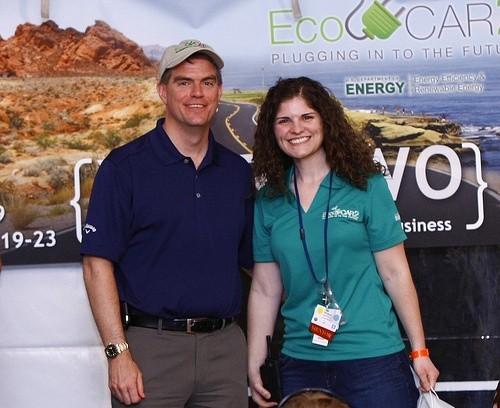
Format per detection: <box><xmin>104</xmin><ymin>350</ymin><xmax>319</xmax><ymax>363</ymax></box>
<box><xmin>155</xmin><ymin>39</ymin><xmax>227</xmax><ymax>82</ymax></box>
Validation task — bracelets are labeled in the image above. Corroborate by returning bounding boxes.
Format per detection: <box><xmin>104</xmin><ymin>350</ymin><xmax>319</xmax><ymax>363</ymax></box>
<box><xmin>410</xmin><ymin>349</ymin><xmax>431</xmax><ymax>360</ymax></box>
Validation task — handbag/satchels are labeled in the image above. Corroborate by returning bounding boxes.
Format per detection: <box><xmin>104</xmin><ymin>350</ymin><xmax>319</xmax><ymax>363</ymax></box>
<box><xmin>409</xmin><ymin>363</ymin><xmax>455</xmax><ymax>408</ymax></box>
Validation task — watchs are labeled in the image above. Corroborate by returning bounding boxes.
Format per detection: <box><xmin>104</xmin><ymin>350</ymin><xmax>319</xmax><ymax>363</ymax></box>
<box><xmin>106</xmin><ymin>339</ymin><xmax>129</xmax><ymax>359</ymax></box>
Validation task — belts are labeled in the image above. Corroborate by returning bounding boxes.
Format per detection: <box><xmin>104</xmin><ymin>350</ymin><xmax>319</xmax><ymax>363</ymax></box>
<box><xmin>117</xmin><ymin>309</ymin><xmax>240</xmax><ymax>335</ymax></box>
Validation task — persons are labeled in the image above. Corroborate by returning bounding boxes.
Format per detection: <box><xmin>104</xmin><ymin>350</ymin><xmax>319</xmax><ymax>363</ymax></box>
<box><xmin>244</xmin><ymin>73</ymin><xmax>441</xmax><ymax>408</ymax></box>
<box><xmin>76</xmin><ymin>39</ymin><xmax>264</xmax><ymax>408</ymax></box>
